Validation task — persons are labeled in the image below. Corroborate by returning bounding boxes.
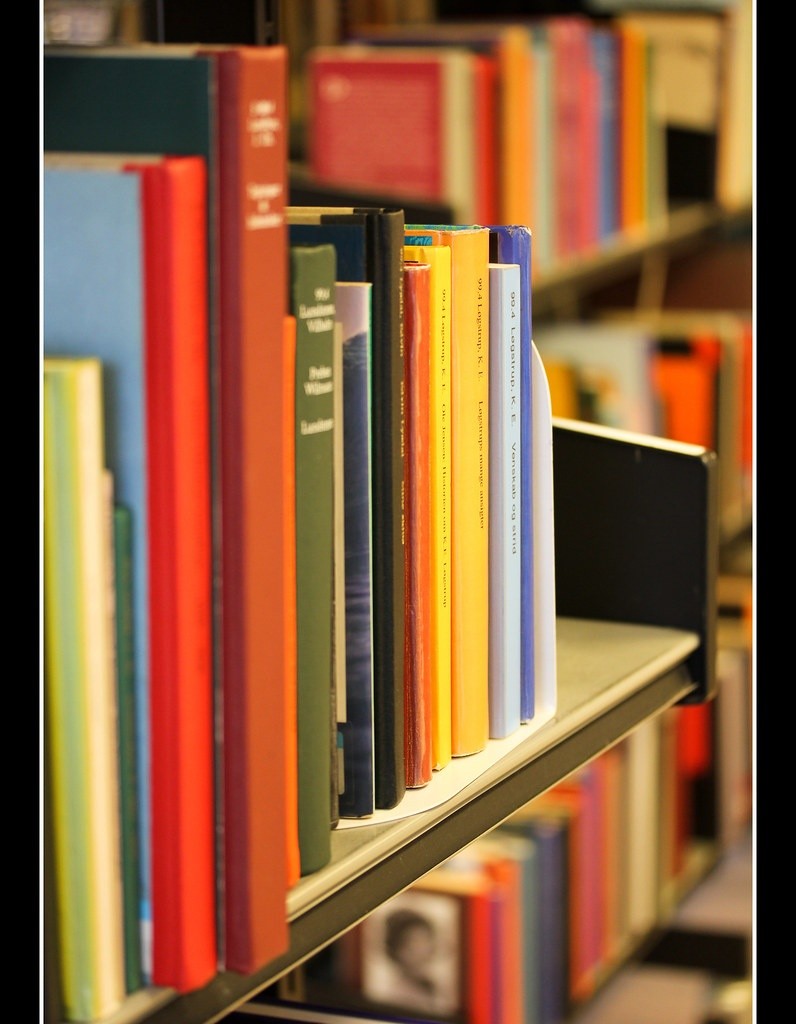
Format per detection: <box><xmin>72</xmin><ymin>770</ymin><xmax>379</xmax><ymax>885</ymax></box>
<box><xmin>366</xmin><ymin>906</ymin><xmax>456</xmax><ymax>1015</ymax></box>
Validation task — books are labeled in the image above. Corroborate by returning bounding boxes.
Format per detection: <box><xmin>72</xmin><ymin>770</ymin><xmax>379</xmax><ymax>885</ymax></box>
<box><xmin>36</xmin><ymin>0</ymin><xmax>754</xmax><ymax>1024</ymax></box>
